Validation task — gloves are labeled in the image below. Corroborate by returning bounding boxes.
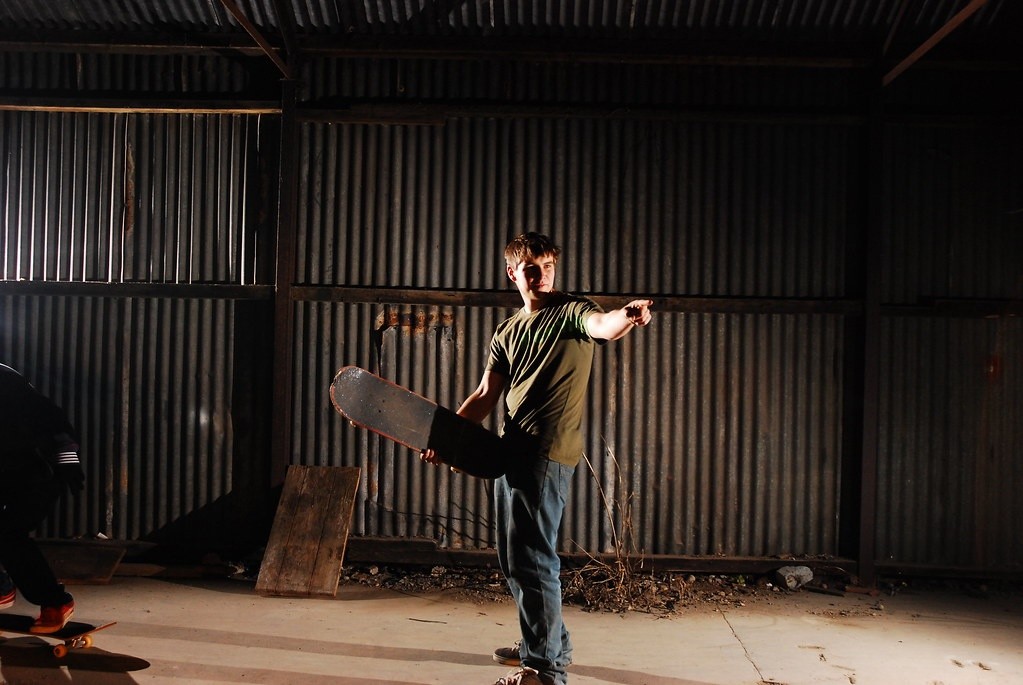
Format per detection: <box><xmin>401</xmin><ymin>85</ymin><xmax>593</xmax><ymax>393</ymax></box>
<box><xmin>63</xmin><ymin>464</ymin><xmax>85</xmax><ymax>495</ymax></box>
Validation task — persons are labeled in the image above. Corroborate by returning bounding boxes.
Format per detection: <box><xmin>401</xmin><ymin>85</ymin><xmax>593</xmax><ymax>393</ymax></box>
<box><xmin>420</xmin><ymin>233</ymin><xmax>653</xmax><ymax>685</ymax></box>
<box><xmin>0</xmin><ymin>362</ymin><xmax>85</xmax><ymax>634</ymax></box>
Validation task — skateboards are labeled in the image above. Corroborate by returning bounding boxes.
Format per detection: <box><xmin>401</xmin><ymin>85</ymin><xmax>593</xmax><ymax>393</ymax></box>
<box><xmin>328</xmin><ymin>364</ymin><xmax>509</xmax><ymax>482</ymax></box>
<box><xmin>0</xmin><ymin>606</ymin><xmax>118</xmax><ymax>659</ymax></box>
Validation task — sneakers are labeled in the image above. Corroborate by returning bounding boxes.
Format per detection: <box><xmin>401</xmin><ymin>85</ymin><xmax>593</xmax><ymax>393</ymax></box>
<box><xmin>30</xmin><ymin>585</ymin><xmax>74</xmax><ymax>634</ymax></box>
<box><xmin>493</xmin><ymin>640</ymin><xmax>572</xmax><ymax>668</ymax></box>
<box><xmin>0</xmin><ymin>570</ymin><xmax>16</xmax><ymax>610</ymax></box>
<box><xmin>493</xmin><ymin>667</ymin><xmax>543</xmax><ymax>685</ymax></box>
